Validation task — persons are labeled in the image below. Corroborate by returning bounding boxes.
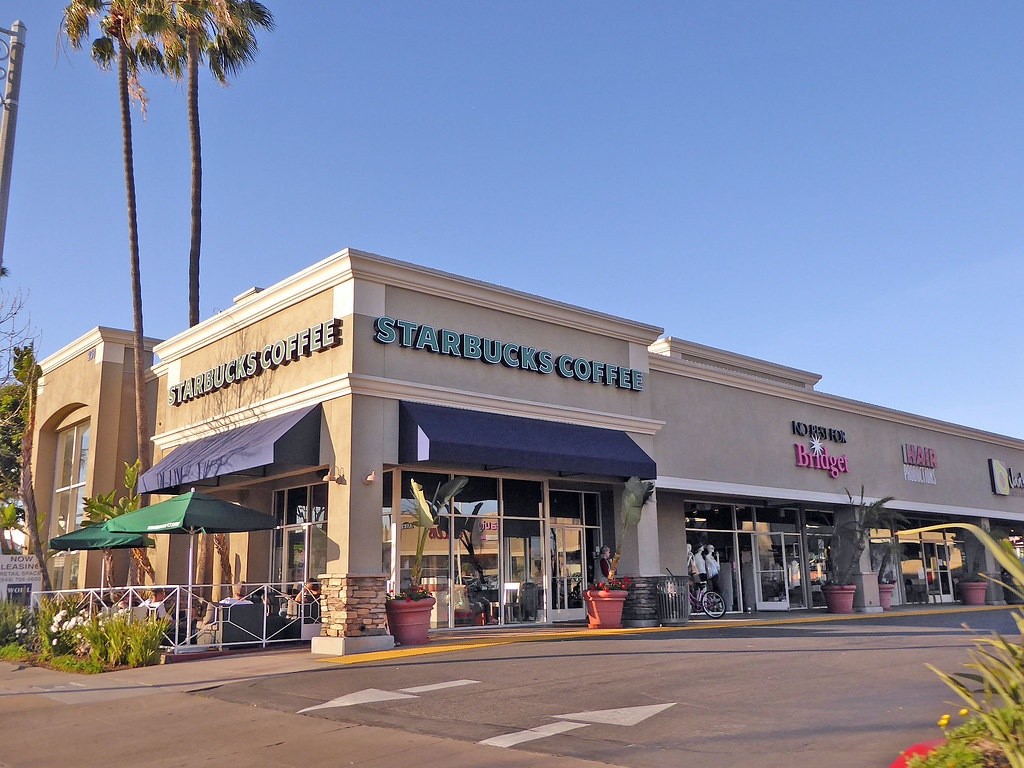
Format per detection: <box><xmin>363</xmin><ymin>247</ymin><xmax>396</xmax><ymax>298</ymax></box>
<box><xmin>218</xmin><ymin>581</ymin><xmax>255</xmax><ymax>605</ymax></box>
<box><xmin>464</xmin><ymin>566</ymin><xmax>499</xmax><ymax>625</ymax></box>
<box><xmin>687</xmin><ymin>544</ymin><xmax>724</xmax><ymax>612</ymax></box>
<box><xmin>285</xmin><ymin>578</ymin><xmax>320</xmax><ymax>620</ymax></box>
<box><xmin>110</xmin><ymin>589</ymin><xmax>130</xmax><ymax>609</ymax></box>
<box><xmin>599</xmin><ymin>545</ymin><xmax>619</xmax><ymax>576</ymax></box>
<box><xmin>191</xmin><ymin>583</ymin><xmax>214</xmax><ymax>645</ymax></box>
<box><xmin>138</xmin><ymin>589</ymin><xmax>174</xmax><ymax>621</ymax></box>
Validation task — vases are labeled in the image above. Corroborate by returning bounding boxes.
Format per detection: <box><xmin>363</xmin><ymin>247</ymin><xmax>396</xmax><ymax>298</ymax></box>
<box><xmin>822</xmin><ymin>583</ymin><xmax>856</xmax><ymax>614</ymax></box>
<box><xmin>385</xmin><ymin>597</ymin><xmax>435</xmax><ymax>645</ymax></box>
<box><xmin>583</xmin><ymin>589</ymin><xmax>628</xmax><ymax>630</ymax></box>
<box><xmin>878</xmin><ymin>583</ymin><xmax>897</xmax><ymax>611</ymax></box>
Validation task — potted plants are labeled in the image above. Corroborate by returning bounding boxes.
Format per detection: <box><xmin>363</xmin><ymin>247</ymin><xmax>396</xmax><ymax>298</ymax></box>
<box><xmin>958</xmin><ymin>517</ymin><xmax>1008</xmax><ymax>605</ymax></box>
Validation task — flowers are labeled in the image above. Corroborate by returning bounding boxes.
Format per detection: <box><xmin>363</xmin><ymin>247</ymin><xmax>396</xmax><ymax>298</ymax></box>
<box><xmin>590</xmin><ymin>476</ymin><xmax>651</xmax><ymax>592</ymax></box>
<box><xmin>823</xmin><ymin>483</ymin><xmax>911</xmax><ymax>585</ymax></box>
<box><xmin>870</xmin><ymin>512</ymin><xmax>910</xmax><ymax>583</ymax></box>
<box><xmin>388</xmin><ymin>476</ymin><xmax>471</xmax><ymax>601</ymax></box>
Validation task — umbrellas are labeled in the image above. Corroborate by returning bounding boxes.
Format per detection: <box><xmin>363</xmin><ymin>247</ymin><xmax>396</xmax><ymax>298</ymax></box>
<box><xmin>50</xmin><ymin>518</ymin><xmax>156</xmax><ymax>600</ymax></box>
<box><xmin>101</xmin><ymin>487</ymin><xmax>279</xmax><ymax>645</ymax></box>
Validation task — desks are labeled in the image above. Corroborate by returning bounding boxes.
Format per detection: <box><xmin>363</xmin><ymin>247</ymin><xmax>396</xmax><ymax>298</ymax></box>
<box><xmin>275</xmin><ymin>594</ymin><xmax>296</xmax><ymax>598</ymax></box>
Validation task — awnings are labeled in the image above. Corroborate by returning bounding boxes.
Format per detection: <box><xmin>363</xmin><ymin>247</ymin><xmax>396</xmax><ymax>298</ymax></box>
<box><xmin>398</xmin><ymin>400</ymin><xmax>657</xmax><ymax>480</ymax></box>
<box><xmin>137</xmin><ymin>402</ymin><xmax>322</xmax><ymax>494</ymax></box>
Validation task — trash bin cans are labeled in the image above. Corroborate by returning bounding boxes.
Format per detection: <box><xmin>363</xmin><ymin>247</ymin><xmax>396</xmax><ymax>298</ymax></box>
<box><xmin>654</xmin><ymin>575</ymin><xmax>692</xmax><ymax>627</ymax></box>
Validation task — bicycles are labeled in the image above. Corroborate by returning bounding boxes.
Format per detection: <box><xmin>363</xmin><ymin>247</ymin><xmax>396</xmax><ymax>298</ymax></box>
<box><xmin>688</xmin><ymin>582</ymin><xmax>726</xmax><ymax>619</ymax></box>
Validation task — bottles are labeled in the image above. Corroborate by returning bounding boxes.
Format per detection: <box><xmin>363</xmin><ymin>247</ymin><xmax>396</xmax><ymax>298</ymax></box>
<box><xmin>748</xmin><ymin>605</ymin><xmax>751</xmax><ymax>615</ymax></box>
<box><xmin>198</xmin><ymin>605</ymin><xmax>202</xmax><ymax>616</ymax></box>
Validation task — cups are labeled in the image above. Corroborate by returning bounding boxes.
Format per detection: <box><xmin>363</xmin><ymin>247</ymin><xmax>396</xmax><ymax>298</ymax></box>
<box><xmin>292</xmin><ymin>589</ymin><xmax>296</xmax><ymax>594</ymax></box>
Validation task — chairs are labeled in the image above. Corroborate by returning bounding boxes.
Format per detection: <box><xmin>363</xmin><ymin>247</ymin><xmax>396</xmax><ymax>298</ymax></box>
<box><xmin>289</xmin><ymin>593</ymin><xmax>321</xmax><ymax>637</ymax></box>
<box><xmin>130</xmin><ymin>608</ymin><xmax>188</xmax><ymax>647</ymax></box>
<box><xmin>908</xmin><ymin>579</ymin><xmax>942</xmax><ymax>606</ymax></box>
<box><xmin>196</xmin><ymin>598</ymin><xmax>264</xmax><ymax>646</ymax></box>
